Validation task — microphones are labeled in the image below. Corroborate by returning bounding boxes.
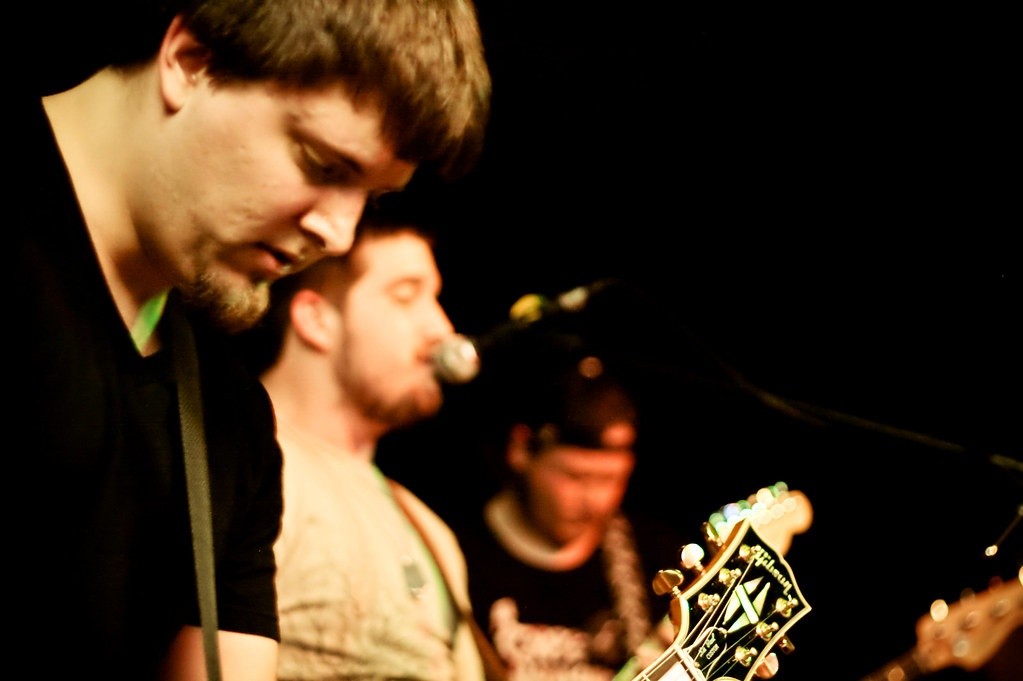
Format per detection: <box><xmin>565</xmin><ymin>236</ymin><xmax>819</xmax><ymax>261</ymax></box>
<box><xmin>435</xmin><ymin>271</ymin><xmax>604</xmax><ymax>385</ymax></box>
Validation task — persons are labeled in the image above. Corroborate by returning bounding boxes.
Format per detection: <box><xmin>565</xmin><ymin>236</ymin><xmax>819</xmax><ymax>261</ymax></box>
<box><xmin>461</xmin><ymin>366</ymin><xmax>674</xmax><ymax>681</ymax></box>
<box><xmin>258</xmin><ymin>212</ymin><xmax>489</xmax><ymax>681</ymax></box>
<box><xmin>0</xmin><ymin>0</ymin><xmax>492</xmax><ymax>681</ymax></box>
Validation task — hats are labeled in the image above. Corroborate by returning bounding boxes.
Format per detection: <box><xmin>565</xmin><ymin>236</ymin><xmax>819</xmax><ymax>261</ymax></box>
<box><xmin>527</xmin><ymin>381</ymin><xmax>638</xmax><ymax>454</ymax></box>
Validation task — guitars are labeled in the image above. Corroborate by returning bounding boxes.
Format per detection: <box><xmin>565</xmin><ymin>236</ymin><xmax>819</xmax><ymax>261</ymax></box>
<box><xmin>868</xmin><ymin>575</ymin><xmax>1023</xmax><ymax>681</ymax></box>
<box><xmin>631</xmin><ymin>517</ymin><xmax>813</xmax><ymax>681</ymax></box>
<box><xmin>617</xmin><ymin>479</ymin><xmax>814</xmax><ymax>681</ymax></box>
